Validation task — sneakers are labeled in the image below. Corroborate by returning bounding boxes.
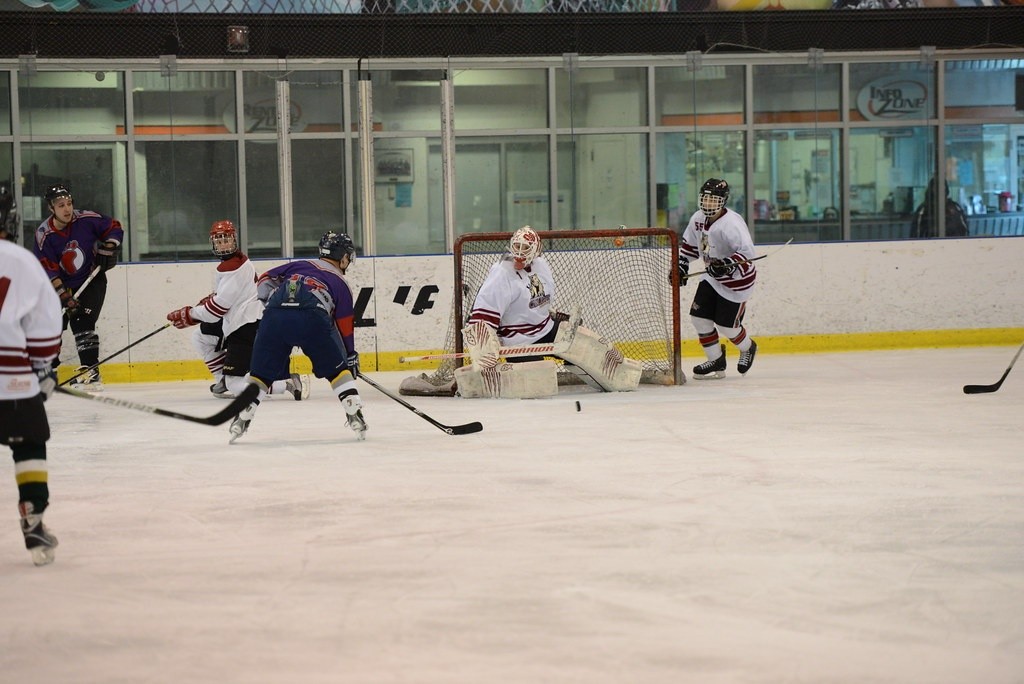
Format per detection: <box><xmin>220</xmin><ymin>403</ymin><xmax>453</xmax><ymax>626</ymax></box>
<box><xmin>286</xmin><ymin>373</ymin><xmax>302</xmax><ymax>400</ymax></box>
<box><xmin>70</xmin><ymin>365</ymin><xmax>99</xmax><ymax>385</ymax></box>
<box><xmin>210</xmin><ymin>375</ymin><xmax>228</xmax><ymax>394</ymax></box>
<box><xmin>229</xmin><ymin>402</ymin><xmax>258</xmax><ymax>435</ymax></box>
<box><xmin>52</xmin><ymin>369</ymin><xmax>58</xmax><ymax>378</ymax></box>
<box><xmin>341</xmin><ymin>394</ymin><xmax>369</xmax><ymax>431</ymax></box>
<box><xmin>737</xmin><ymin>338</ymin><xmax>757</xmax><ymax>373</ymax></box>
<box><xmin>693</xmin><ymin>344</ymin><xmax>726</xmax><ymax>374</ymax></box>
<box><xmin>20</xmin><ymin>512</ymin><xmax>58</xmax><ymax>550</ymax></box>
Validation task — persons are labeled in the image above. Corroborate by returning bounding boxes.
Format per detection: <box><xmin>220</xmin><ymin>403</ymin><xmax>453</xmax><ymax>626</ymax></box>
<box><xmin>0</xmin><ymin>182</ymin><xmax>63</xmax><ymax>567</ymax></box>
<box><xmin>909</xmin><ymin>177</ymin><xmax>970</xmax><ymax>239</ymax></box>
<box><xmin>167</xmin><ymin>221</ymin><xmax>311</xmax><ymax>402</ymax></box>
<box><xmin>34</xmin><ymin>186</ymin><xmax>124</xmax><ymax>386</ymax></box>
<box><xmin>469</xmin><ymin>225</ymin><xmax>643</xmax><ymax>390</ymax></box>
<box><xmin>668</xmin><ymin>179</ymin><xmax>757</xmax><ymax>380</ymax></box>
<box><xmin>231</xmin><ymin>231</ymin><xmax>369</xmax><ymax>438</ymax></box>
<box><xmin>819</xmin><ymin>207</ymin><xmax>842</xmax><ymax>242</ymax></box>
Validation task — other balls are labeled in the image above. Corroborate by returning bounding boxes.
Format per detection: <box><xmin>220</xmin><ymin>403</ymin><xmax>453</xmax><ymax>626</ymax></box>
<box><xmin>576</xmin><ymin>401</ymin><xmax>581</xmax><ymax>412</ymax></box>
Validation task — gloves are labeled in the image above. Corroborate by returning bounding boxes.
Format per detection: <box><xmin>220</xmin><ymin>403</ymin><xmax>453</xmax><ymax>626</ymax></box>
<box><xmin>668</xmin><ymin>255</ymin><xmax>689</xmax><ymax>286</ymax></box>
<box><xmin>556</xmin><ymin>312</ymin><xmax>582</xmax><ymax>326</ymax></box>
<box><xmin>55</xmin><ymin>283</ymin><xmax>79</xmax><ymax>311</ymax></box>
<box><xmin>167</xmin><ymin>307</ymin><xmax>198</xmax><ymax>328</ymax></box>
<box><xmin>705</xmin><ymin>256</ymin><xmax>738</xmax><ymax>278</ymax></box>
<box><xmin>196</xmin><ymin>293</ymin><xmax>217</xmax><ymax>306</ymax></box>
<box><xmin>32</xmin><ymin>360</ymin><xmax>58</xmax><ymax>401</ymax></box>
<box><xmin>348</xmin><ymin>350</ymin><xmax>360</xmax><ymax>379</ymax></box>
<box><xmin>95</xmin><ymin>242</ymin><xmax>119</xmax><ymax>271</ymax></box>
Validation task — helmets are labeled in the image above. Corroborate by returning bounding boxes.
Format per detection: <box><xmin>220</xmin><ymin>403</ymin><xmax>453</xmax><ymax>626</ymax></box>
<box><xmin>510</xmin><ymin>227</ymin><xmax>542</xmax><ymax>270</ymax></box>
<box><xmin>209</xmin><ymin>220</ymin><xmax>238</xmax><ymax>255</ymax></box>
<box><xmin>46</xmin><ymin>184</ymin><xmax>75</xmax><ymax>208</ymax></box>
<box><xmin>0</xmin><ymin>185</ymin><xmax>20</xmax><ymax>244</ymax></box>
<box><xmin>698</xmin><ymin>177</ymin><xmax>730</xmax><ymax>217</ymax></box>
<box><xmin>319</xmin><ymin>230</ymin><xmax>356</xmax><ymax>275</ymax></box>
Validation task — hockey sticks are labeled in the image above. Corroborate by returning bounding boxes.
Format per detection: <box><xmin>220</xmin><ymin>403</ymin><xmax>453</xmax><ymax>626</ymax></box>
<box><xmin>59</xmin><ymin>320</ymin><xmax>176</xmax><ymax>387</ymax></box>
<box><xmin>62</xmin><ymin>265</ymin><xmax>102</xmax><ymax>315</ymax></box>
<box><xmin>962</xmin><ymin>345</ymin><xmax>1024</xmax><ymax>394</ymax></box>
<box><xmin>399</xmin><ymin>302</ymin><xmax>584</xmax><ymax>364</ymax></box>
<box><xmin>356</xmin><ymin>371</ymin><xmax>483</xmax><ymax>436</ymax></box>
<box><xmin>683</xmin><ymin>238</ymin><xmax>794</xmax><ymax>279</ymax></box>
<box><xmin>51</xmin><ymin>382</ymin><xmax>260</xmax><ymax>427</ymax></box>
